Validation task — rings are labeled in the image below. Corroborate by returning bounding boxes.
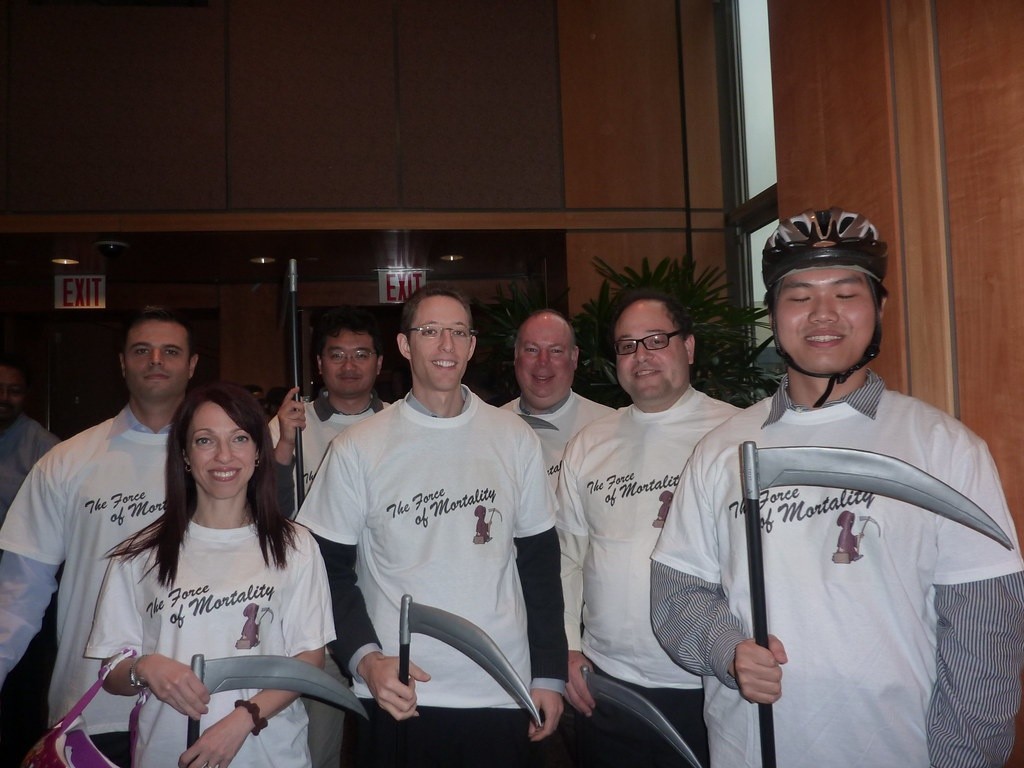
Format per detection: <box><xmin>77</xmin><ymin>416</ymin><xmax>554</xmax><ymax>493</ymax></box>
<box><xmin>208</xmin><ymin>764</ymin><xmax>213</xmax><ymax>768</ymax></box>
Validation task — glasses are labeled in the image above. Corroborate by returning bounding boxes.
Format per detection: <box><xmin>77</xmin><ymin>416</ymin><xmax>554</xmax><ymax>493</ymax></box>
<box><xmin>321</xmin><ymin>348</ymin><xmax>378</xmax><ymax>363</ymax></box>
<box><xmin>613</xmin><ymin>327</ymin><xmax>683</xmax><ymax>355</ymax></box>
<box><xmin>406</xmin><ymin>324</ymin><xmax>477</xmax><ymax>343</ymax></box>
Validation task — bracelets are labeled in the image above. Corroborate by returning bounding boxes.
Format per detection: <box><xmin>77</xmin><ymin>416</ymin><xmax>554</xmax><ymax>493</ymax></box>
<box><xmin>130</xmin><ymin>655</ymin><xmax>148</xmax><ymax>688</ymax></box>
<box><xmin>235</xmin><ymin>700</ymin><xmax>268</xmax><ymax>736</ymax></box>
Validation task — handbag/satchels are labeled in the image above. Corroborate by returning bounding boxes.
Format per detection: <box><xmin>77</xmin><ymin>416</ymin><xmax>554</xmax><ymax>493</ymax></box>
<box><xmin>21</xmin><ymin>646</ymin><xmax>151</xmax><ymax>768</ymax></box>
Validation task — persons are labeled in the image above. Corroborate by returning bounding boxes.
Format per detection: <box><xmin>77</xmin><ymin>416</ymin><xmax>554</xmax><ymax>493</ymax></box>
<box><xmin>266</xmin><ymin>307</ymin><xmax>394</xmax><ymax>525</ymax></box>
<box><xmin>554</xmin><ymin>288</ymin><xmax>747</xmax><ymax>768</ymax></box>
<box><xmin>0</xmin><ymin>358</ymin><xmax>63</xmax><ymax>529</ymax></box>
<box><xmin>82</xmin><ymin>383</ymin><xmax>337</xmax><ymax>768</ymax></box>
<box><xmin>0</xmin><ymin>306</ymin><xmax>198</xmax><ymax>768</ymax></box>
<box><xmin>293</xmin><ymin>285</ymin><xmax>569</xmax><ymax>768</ymax></box>
<box><xmin>498</xmin><ymin>309</ymin><xmax>618</xmax><ymax>495</ymax></box>
<box><xmin>648</xmin><ymin>206</ymin><xmax>1023</xmax><ymax>768</ymax></box>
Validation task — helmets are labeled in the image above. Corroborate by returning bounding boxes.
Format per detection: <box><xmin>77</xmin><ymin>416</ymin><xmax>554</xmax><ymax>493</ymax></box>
<box><xmin>761</xmin><ymin>205</ymin><xmax>889</xmax><ymax>291</ymax></box>
